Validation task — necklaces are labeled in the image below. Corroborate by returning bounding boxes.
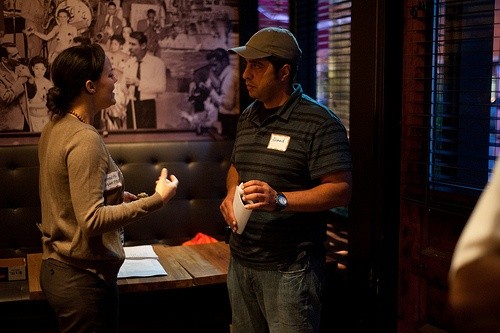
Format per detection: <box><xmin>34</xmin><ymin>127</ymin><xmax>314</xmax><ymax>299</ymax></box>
<box><xmin>67</xmin><ymin>110</ymin><xmax>91</xmax><ymax>125</ymax></box>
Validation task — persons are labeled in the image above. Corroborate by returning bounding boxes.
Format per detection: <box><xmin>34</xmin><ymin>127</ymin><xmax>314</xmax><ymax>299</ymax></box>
<box><xmin>219</xmin><ymin>27</ymin><xmax>353</xmax><ymax>333</ymax></box>
<box><xmin>450</xmin><ymin>154</ymin><xmax>500</xmax><ymax>310</ymax></box>
<box><xmin>38</xmin><ymin>42</ymin><xmax>179</xmax><ymax>333</ymax></box>
<box><xmin>0</xmin><ymin>0</ymin><xmax>240</xmax><ymax>139</ymax></box>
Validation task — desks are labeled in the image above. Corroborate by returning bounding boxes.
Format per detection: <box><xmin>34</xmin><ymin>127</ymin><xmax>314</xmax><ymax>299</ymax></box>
<box><xmin>27</xmin><ymin>244</ymin><xmax>193</xmax><ymax>333</ymax></box>
<box><xmin>172</xmin><ymin>243</ymin><xmax>227</xmax><ymax>288</ymax></box>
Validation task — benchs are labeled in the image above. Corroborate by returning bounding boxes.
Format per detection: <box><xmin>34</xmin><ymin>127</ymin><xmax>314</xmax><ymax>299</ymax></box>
<box><xmin>0</xmin><ymin>140</ymin><xmax>234</xmax><ymax>333</ymax></box>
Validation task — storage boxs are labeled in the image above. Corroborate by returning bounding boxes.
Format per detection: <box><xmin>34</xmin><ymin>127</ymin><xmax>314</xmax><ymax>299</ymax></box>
<box><xmin>0</xmin><ymin>257</ymin><xmax>26</xmax><ymax>281</ymax></box>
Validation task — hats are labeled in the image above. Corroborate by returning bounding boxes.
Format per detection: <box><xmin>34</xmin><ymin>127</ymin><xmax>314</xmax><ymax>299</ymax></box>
<box><xmin>230</xmin><ymin>26</ymin><xmax>303</xmax><ymax>62</ymax></box>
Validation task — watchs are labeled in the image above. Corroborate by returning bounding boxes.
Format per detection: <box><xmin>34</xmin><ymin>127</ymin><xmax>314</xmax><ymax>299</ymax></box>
<box><xmin>273</xmin><ymin>190</ymin><xmax>289</xmax><ymax>213</ymax></box>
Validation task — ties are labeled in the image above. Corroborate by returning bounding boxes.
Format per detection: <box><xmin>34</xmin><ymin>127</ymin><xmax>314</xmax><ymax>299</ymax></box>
<box><xmin>105</xmin><ymin>15</ymin><xmax>111</xmax><ymax>30</ymax></box>
<box><xmin>135</xmin><ymin>61</ymin><xmax>141</xmax><ymax>99</ymax></box>
